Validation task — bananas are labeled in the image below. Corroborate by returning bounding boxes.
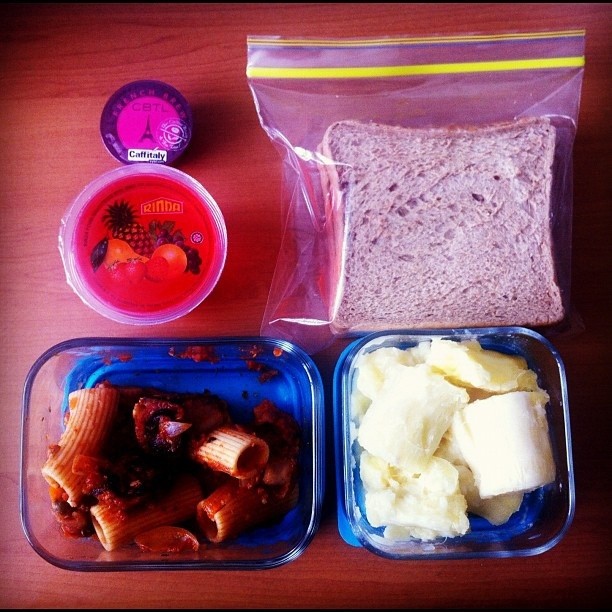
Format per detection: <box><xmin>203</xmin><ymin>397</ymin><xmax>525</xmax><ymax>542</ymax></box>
<box><xmin>357</xmin><ymin>339</ymin><xmax>557</xmax><ymax>546</ymax></box>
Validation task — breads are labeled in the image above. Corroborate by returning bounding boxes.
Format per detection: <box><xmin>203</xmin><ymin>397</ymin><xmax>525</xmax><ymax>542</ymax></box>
<box><xmin>315</xmin><ymin>115</ymin><xmax>567</xmax><ymax>337</ymax></box>
